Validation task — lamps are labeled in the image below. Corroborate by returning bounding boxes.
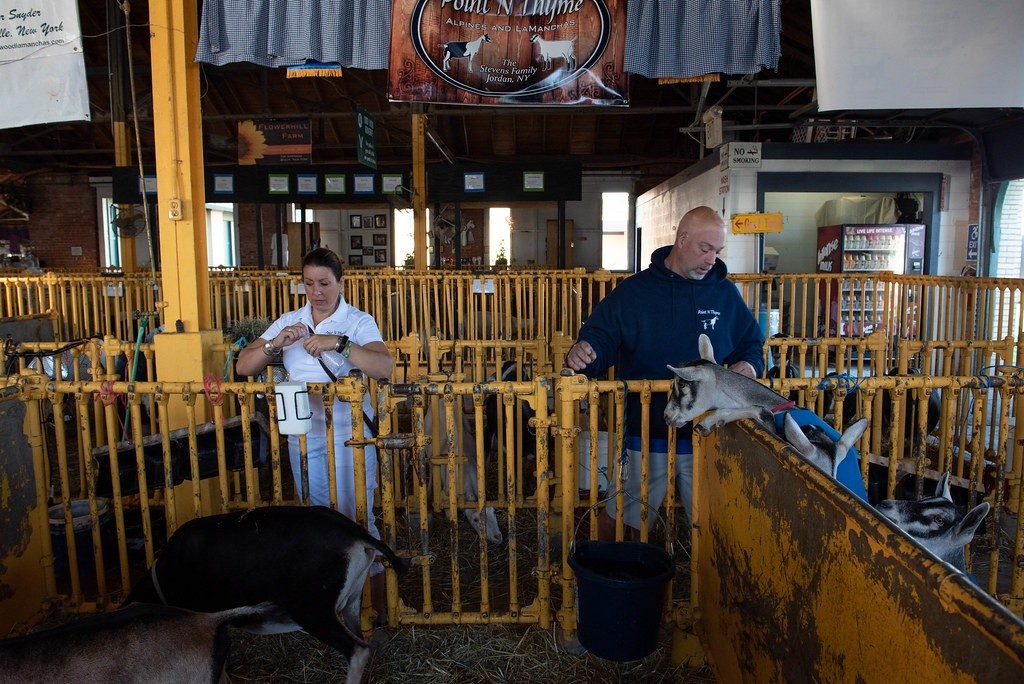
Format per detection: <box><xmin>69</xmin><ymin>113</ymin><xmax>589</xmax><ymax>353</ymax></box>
<box><xmin>702</xmin><ymin>106</ymin><xmax>723</xmax><ymax>149</ymax></box>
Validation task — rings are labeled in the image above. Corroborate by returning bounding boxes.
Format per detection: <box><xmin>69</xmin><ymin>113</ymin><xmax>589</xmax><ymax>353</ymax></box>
<box><xmin>312</xmin><ymin>347</ymin><xmax>316</xmax><ymax>350</ymax></box>
<box><xmin>289</xmin><ymin>328</ymin><xmax>291</xmax><ymax>331</ymax></box>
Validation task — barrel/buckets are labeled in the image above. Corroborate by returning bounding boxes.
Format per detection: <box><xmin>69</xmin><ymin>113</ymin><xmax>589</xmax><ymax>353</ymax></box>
<box><xmin>883</xmin><ymin>366</ymin><xmax>941</xmax><ymax>440</ymax></box>
<box><xmin>1005</xmin><ymin>417</ymin><xmax>1016</xmax><ymax>472</ymax></box>
<box><xmin>47</xmin><ymin>499</ymin><xmax>110</xmax><ymax>593</ymax></box>
<box><xmin>567</xmin><ymin>488</ymin><xmax>676</xmax><ymax>663</ymax></box>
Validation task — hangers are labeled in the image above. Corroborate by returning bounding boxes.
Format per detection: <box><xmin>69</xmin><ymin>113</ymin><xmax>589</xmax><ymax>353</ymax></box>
<box><xmin>0</xmin><ymin>193</ymin><xmax>30</xmax><ymax>221</ymax></box>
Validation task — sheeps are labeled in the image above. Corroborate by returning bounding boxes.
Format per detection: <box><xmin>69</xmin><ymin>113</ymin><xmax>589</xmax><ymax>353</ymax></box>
<box><xmin>423</xmin><ymin>399</ymin><xmax>503</xmax><ymax>545</ymax></box>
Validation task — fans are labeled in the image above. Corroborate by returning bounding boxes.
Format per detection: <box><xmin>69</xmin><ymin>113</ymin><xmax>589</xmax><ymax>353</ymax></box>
<box><xmin>110</xmin><ymin>205</ymin><xmax>146</xmax><ymax>238</ymax></box>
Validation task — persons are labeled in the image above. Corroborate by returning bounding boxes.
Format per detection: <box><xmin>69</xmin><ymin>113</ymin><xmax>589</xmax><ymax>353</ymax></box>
<box><xmin>235</xmin><ymin>247</ymin><xmax>394</xmax><ymax>612</ymax></box>
<box><xmin>565</xmin><ymin>205</ymin><xmax>766</xmax><ymax>547</ymax></box>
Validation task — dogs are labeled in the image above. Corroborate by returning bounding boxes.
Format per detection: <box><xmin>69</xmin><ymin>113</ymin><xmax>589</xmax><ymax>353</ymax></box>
<box><xmin>662</xmin><ymin>336</ymin><xmax>870</xmax><ymax>501</ymax></box>
<box><xmin>118</xmin><ymin>504</ymin><xmax>407</xmax><ymax>684</ymax></box>
<box><xmin>874</xmin><ymin>470</ymin><xmax>991</xmax><ymax>574</ymax></box>
<box><xmin>784</xmin><ymin>409</ymin><xmax>868</xmax><ymax>479</ymax></box>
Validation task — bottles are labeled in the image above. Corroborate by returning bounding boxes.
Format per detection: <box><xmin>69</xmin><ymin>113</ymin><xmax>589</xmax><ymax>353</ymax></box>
<box><xmin>300</xmin><ymin>328</ymin><xmax>347</xmax><ymax>374</ymax></box>
<box><xmin>841</xmin><ymin>234</ymin><xmax>898</xmax><ymax>323</ymax></box>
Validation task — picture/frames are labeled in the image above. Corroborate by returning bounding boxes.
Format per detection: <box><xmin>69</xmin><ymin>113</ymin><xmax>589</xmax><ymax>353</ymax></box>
<box><xmin>350</xmin><ymin>235</ymin><xmax>363</xmax><ymax>249</ymax></box>
<box><xmin>374</xmin><ymin>214</ymin><xmax>386</xmax><ymax>228</ymax></box>
<box><xmin>375</xmin><ymin>249</ymin><xmax>387</xmax><ymax>263</ymax></box>
<box><xmin>373</xmin><ymin>234</ymin><xmax>386</xmax><ymax>246</ymax></box>
<box><xmin>350</xmin><ymin>214</ymin><xmax>362</xmax><ymax>229</ymax></box>
<box><xmin>349</xmin><ymin>255</ymin><xmax>363</xmax><ymax>265</ymax></box>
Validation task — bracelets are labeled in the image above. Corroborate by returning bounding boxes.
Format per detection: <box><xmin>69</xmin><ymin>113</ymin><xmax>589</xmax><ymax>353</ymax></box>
<box><xmin>262</xmin><ymin>338</ymin><xmax>281</xmax><ymax>357</ymax></box>
<box><xmin>335</xmin><ymin>335</ymin><xmax>353</xmax><ymax>358</ymax></box>
